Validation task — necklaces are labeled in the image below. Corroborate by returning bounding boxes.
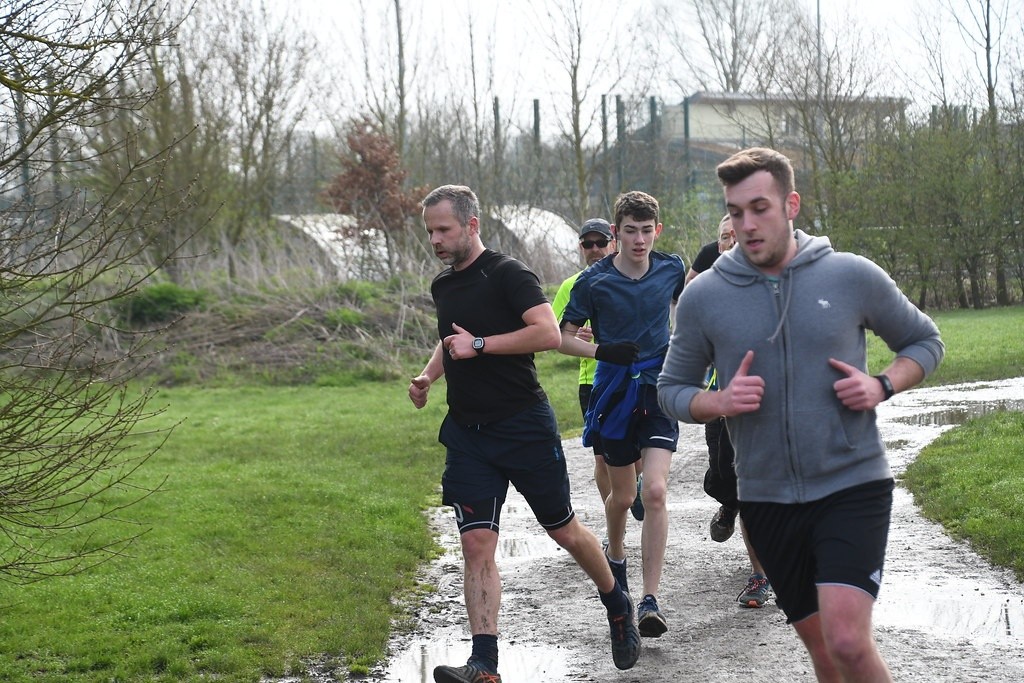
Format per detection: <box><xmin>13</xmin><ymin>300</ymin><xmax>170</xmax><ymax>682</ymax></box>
<box><xmin>616</xmin><ymin>266</ymin><xmax>648</xmax><ymax>281</ymax></box>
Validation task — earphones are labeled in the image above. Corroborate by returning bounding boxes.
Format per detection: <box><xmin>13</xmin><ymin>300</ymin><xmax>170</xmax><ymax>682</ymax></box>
<box><xmin>615</xmin><ymin>229</ymin><xmax>617</xmax><ymax>237</ymax></box>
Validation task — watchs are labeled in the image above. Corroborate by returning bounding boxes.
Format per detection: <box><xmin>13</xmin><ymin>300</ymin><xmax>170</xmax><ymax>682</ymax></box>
<box><xmin>471</xmin><ymin>337</ymin><xmax>485</xmax><ymax>357</ymax></box>
<box><xmin>873</xmin><ymin>374</ymin><xmax>895</xmax><ymax>401</ymax></box>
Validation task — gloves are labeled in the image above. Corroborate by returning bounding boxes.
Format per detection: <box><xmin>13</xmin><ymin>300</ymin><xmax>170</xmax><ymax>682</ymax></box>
<box><xmin>595</xmin><ymin>340</ymin><xmax>641</xmax><ymax>367</ymax></box>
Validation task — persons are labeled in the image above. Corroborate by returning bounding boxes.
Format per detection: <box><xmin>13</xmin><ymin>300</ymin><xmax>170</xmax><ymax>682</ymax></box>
<box><xmin>681</xmin><ymin>209</ymin><xmax>773</xmax><ymax>608</ymax></box>
<box><xmin>655</xmin><ymin>146</ymin><xmax>948</xmax><ymax>683</ymax></box>
<box><xmin>556</xmin><ymin>190</ymin><xmax>685</xmax><ymax>637</ymax></box>
<box><xmin>406</xmin><ymin>182</ymin><xmax>641</xmax><ymax>683</ymax></box>
<box><xmin>552</xmin><ymin>217</ymin><xmax>644</xmax><ymax>557</ymax></box>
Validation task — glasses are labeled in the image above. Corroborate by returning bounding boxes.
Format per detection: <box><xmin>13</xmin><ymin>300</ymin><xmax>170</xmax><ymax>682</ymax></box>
<box><xmin>581</xmin><ymin>239</ymin><xmax>611</xmax><ymax>250</ymax></box>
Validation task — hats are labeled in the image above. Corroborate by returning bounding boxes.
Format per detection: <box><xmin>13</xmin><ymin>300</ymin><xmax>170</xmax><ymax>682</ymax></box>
<box><xmin>578</xmin><ymin>217</ymin><xmax>614</xmax><ymax>241</ymax></box>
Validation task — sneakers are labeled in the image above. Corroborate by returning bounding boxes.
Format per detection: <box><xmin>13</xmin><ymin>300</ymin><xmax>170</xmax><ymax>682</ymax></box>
<box><xmin>736</xmin><ymin>571</ymin><xmax>770</xmax><ymax>609</ymax></box>
<box><xmin>637</xmin><ymin>594</ymin><xmax>669</xmax><ymax>638</ymax></box>
<box><xmin>631</xmin><ymin>474</ymin><xmax>645</xmax><ymax>521</ymax></box>
<box><xmin>607</xmin><ymin>590</ymin><xmax>642</xmax><ymax>670</ymax></box>
<box><xmin>604</xmin><ymin>543</ymin><xmax>629</xmax><ymax>595</ymax></box>
<box><xmin>433</xmin><ymin>654</ymin><xmax>503</xmax><ymax>683</ymax></box>
<box><xmin>709</xmin><ymin>504</ymin><xmax>739</xmax><ymax>543</ymax></box>
